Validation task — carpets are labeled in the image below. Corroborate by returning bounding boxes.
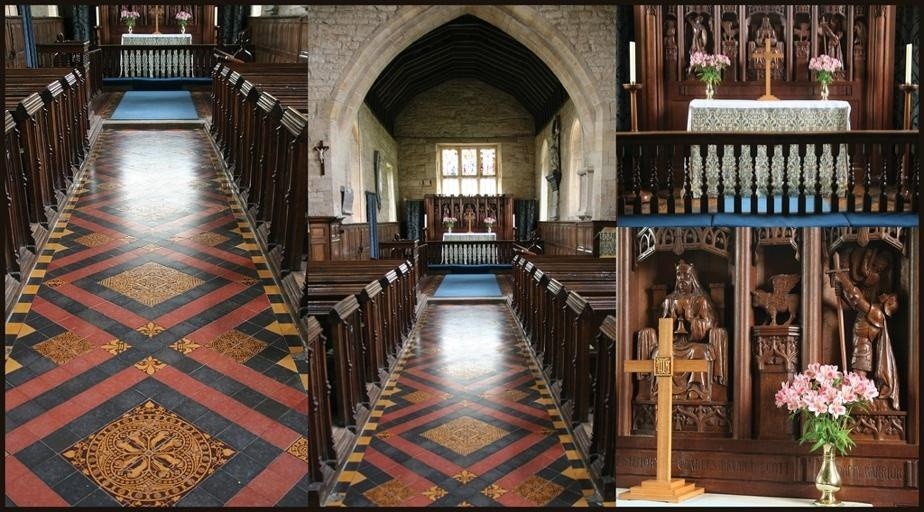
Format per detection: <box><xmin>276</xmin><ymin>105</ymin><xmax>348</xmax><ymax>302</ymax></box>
<box><xmin>434</xmin><ymin>272</ymin><xmax>503</xmax><ymax>298</ymax></box>
<box><xmin>721</xmin><ymin>193</ymin><xmax>831</xmax><ymax>213</ymax></box>
<box><xmin>111</xmin><ymin>90</ymin><xmax>199</xmax><ymax>120</ymax></box>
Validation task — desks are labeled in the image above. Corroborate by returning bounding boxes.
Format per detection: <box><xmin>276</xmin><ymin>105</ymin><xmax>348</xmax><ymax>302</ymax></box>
<box><xmin>688</xmin><ymin>97</ymin><xmax>861</xmax><ymax>199</ymax></box>
<box><xmin>442</xmin><ymin>233</ymin><xmax>498</xmax><ymax>264</ymax></box>
<box><xmin>617</xmin><ymin>488</ymin><xmax>873</xmax><ymax>510</ymax></box>
<box><xmin>121</xmin><ymin>33</ymin><xmax>194</xmax><ymax>77</ymax></box>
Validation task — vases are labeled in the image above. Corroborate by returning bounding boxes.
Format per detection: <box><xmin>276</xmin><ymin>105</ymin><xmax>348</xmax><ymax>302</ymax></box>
<box><xmin>821</xmin><ymin>81</ymin><xmax>829</xmax><ymax>100</ymax></box>
<box><xmin>181</xmin><ymin>25</ymin><xmax>185</xmax><ymax>33</ymax></box>
<box><xmin>488</xmin><ymin>227</ymin><xmax>491</xmax><ymax>233</ymax></box>
<box><xmin>705</xmin><ymin>77</ymin><xmax>714</xmax><ymax>99</ymax></box>
<box><xmin>128</xmin><ymin>24</ymin><xmax>133</xmax><ymax>33</ymax></box>
<box><xmin>815</xmin><ymin>440</ymin><xmax>843</xmax><ymax>504</ymax></box>
<box><xmin>448</xmin><ymin>227</ymin><xmax>452</xmax><ymax>233</ymax></box>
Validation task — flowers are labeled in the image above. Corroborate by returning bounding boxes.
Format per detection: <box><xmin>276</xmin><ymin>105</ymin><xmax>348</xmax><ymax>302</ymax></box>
<box><xmin>690</xmin><ymin>50</ymin><xmax>731</xmax><ymax>87</ymax></box>
<box><xmin>120</xmin><ymin>10</ymin><xmax>140</xmax><ymax>26</ymax></box>
<box><xmin>484</xmin><ymin>217</ymin><xmax>496</xmax><ymax>226</ymax></box>
<box><xmin>176</xmin><ymin>10</ymin><xmax>192</xmax><ymax>26</ymax></box>
<box><xmin>443</xmin><ymin>216</ymin><xmax>457</xmax><ymax>228</ymax></box>
<box><xmin>774</xmin><ymin>362</ymin><xmax>881</xmax><ymax>456</ymax></box>
<box><xmin>808</xmin><ymin>53</ymin><xmax>845</xmax><ymax>87</ymax></box>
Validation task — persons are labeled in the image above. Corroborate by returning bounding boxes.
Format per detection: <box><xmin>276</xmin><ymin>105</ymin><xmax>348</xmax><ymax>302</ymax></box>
<box><xmin>687</xmin><ymin>16</ymin><xmax>707</xmax><ymax>75</ymax></box>
<box><xmin>823</xmin><ymin>16</ymin><xmax>845</xmax><ymax>71</ymax></box>
<box><xmin>652</xmin><ymin>258</ymin><xmax>719</xmax><ymax>399</ymax></box>
<box><xmin>316</xmin><ymin>147</ymin><xmax>327</xmax><ymax>164</ymax></box>
<box><xmin>835</xmin><ymin>271</ymin><xmax>901</xmax><ymax>411</ymax></box>
<box><xmin>756</xmin><ymin>17</ymin><xmax>778</xmax><ymax>66</ymax></box>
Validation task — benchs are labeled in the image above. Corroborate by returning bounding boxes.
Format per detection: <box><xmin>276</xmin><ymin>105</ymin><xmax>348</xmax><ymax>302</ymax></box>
<box><xmin>307</xmin><ymin>257</ymin><xmax>415</xmax><ymax>507</ymax></box>
<box><xmin>2</xmin><ymin>66</ymin><xmax>92</xmax><ymax>322</ymax></box>
<box><xmin>211</xmin><ymin>59</ymin><xmax>306</xmax><ymax>345</ymax></box>
<box><xmin>510</xmin><ymin>252</ymin><xmax>614</xmax><ymax>500</ymax></box>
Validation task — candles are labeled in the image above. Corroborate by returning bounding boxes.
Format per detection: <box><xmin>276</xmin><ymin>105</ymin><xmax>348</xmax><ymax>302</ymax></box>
<box><xmin>214</xmin><ymin>6</ymin><xmax>218</xmax><ymax>26</ymax></box>
<box><xmin>905</xmin><ymin>42</ymin><xmax>913</xmax><ymax>85</ymax></box>
<box><xmin>425</xmin><ymin>214</ymin><xmax>427</xmax><ymax>227</ymax></box>
<box><xmin>96</xmin><ymin>6</ymin><xmax>99</xmax><ymax>26</ymax></box>
<box><xmin>629</xmin><ymin>40</ymin><xmax>636</xmax><ymax>84</ymax></box>
<box><xmin>513</xmin><ymin>214</ymin><xmax>515</xmax><ymax>227</ymax></box>
